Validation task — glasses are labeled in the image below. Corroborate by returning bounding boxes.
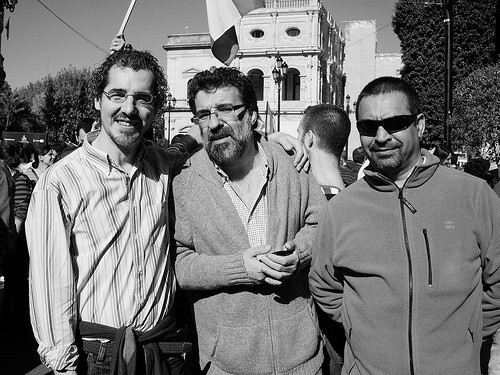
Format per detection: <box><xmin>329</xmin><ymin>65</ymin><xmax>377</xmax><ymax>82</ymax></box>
<box><xmin>191</xmin><ymin>104</ymin><xmax>244</xmax><ymax>125</ymax></box>
<box><xmin>102</xmin><ymin>89</ymin><xmax>158</xmax><ymax>104</ymax></box>
<box><xmin>356</xmin><ymin>115</ymin><xmax>417</xmax><ymax>137</ymax></box>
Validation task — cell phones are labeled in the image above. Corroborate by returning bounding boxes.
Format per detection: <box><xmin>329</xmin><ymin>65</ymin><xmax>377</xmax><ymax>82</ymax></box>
<box><xmin>258</xmin><ymin>250</ymin><xmax>293</xmax><ymax>263</ymax></box>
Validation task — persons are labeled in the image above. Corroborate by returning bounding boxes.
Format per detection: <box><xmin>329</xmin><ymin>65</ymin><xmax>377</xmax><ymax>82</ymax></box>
<box><xmin>170</xmin><ymin>67</ymin><xmax>324</xmax><ymax>375</ymax></box>
<box><xmin>25</xmin><ymin>50</ymin><xmax>310</xmax><ymax>375</ymax></box>
<box><xmin>296</xmin><ymin>105</ymin><xmax>371</xmax><ymax>202</ymax></box>
<box><xmin>0</xmin><ymin>117</ymin><xmax>92</xmax><ymax>314</ymax></box>
<box><xmin>431</xmin><ymin>144</ymin><xmax>500</xmax><ymax>197</ymax></box>
<box><xmin>308</xmin><ymin>76</ymin><xmax>500</xmax><ymax>375</ymax></box>
